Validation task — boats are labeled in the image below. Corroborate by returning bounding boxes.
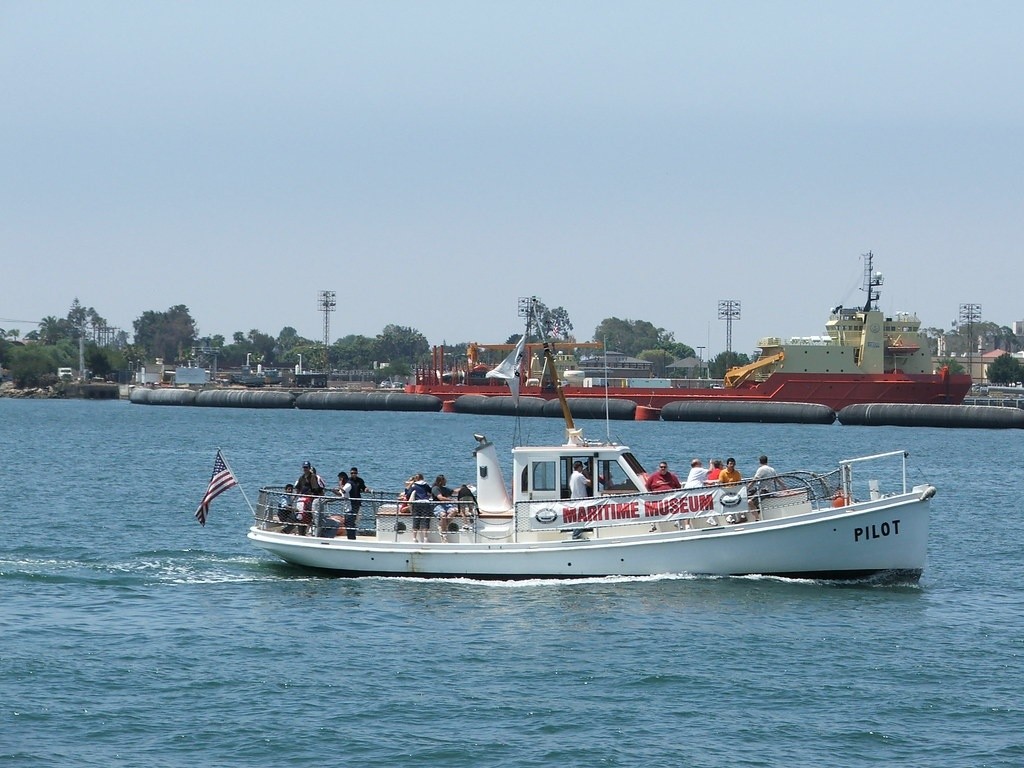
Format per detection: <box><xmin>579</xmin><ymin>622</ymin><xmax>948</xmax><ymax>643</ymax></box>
<box><xmin>404</xmin><ymin>250</ymin><xmax>974</xmax><ymax>411</ymax></box>
<box><xmin>246</xmin><ymin>305</ymin><xmax>936</xmax><ymax>587</ymax></box>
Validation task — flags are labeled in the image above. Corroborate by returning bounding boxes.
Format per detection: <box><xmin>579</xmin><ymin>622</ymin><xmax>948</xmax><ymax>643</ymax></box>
<box><xmin>194</xmin><ymin>454</ymin><xmax>237</xmax><ymax>527</ymax></box>
<box><xmin>485</xmin><ymin>334</ymin><xmax>526</xmax><ymax>409</ymax></box>
<box><xmin>553</xmin><ymin>318</ymin><xmax>558</xmax><ymax>337</ymax></box>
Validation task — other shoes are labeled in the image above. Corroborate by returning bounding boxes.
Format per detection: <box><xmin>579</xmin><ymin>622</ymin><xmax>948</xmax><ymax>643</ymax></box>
<box><xmin>281</xmin><ymin>530</ymin><xmax>286</xmax><ymax>533</ymax></box>
<box><xmin>735</xmin><ymin>517</ymin><xmax>748</xmax><ymax>523</ymax></box>
<box><xmin>685</xmin><ymin>524</ymin><xmax>690</xmax><ymax>529</ymax></box>
<box><xmin>726</xmin><ymin>516</ymin><xmax>736</xmax><ymax>523</ymax></box>
<box><xmin>674</xmin><ymin>521</ymin><xmax>683</xmax><ymax>528</ymax></box>
<box><xmin>470</xmin><ymin>515</ymin><xmax>474</xmax><ymax>523</ymax></box>
<box><xmin>707</xmin><ymin>518</ymin><xmax>718</xmax><ymax>525</ymax></box>
<box><xmin>649</xmin><ymin>526</ymin><xmax>656</xmax><ymax>532</ymax></box>
<box><xmin>463</xmin><ymin>525</ymin><xmax>469</xmax><ymax>530</ymax></box>
<box><xmin>439</xmin><ymin>530</ymin><xmax>448</xmax><ymax>543</ymax></box>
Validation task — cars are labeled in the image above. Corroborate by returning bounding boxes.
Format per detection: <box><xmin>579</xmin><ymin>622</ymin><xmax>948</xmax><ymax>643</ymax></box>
<box><xmin>392</xmin><ymin>382</ymin><xmax>403</xmax><ymax>388</ymax></box>
<box><xmin>381</xmin><ymin>381</ymin><xmax>393</xmax><ymax>388</ymax></box>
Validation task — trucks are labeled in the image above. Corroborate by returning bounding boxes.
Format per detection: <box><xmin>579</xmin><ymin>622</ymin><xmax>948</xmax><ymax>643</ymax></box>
<box><xmin>57</xmin><ymin>367</ymin><xmax>73</xmax><ymax>379</ymax></box>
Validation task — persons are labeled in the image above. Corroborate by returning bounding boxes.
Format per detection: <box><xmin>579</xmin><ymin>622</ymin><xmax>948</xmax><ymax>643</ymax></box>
<box><xmin>310</xmin><ymin>466</ymin><xmax>325</xmax><ymax>487</ymax></box>
<box><xmin>278</xmin><ymin>484</ymin><xmax>299</xmax><ymax>533</ymax></box>
<box><xmin>348</xmin><ymin>468</ymin><xmax>368</xmax><ymax>513</ymax></box>
<box><xmin>736</xmin><ymin>456</ymin><xmax>786</xmax><ymax>523</ymax></box>
<box><xmin>583</xmin><ymin>459</ymin><xmax>604</xmax><ymax>486</ymax></box>
<box><xmin>570</xmin><ymin>461</ymin><xmax>592</xmax><ymax>539</ymax></box>
<box><xmin>645</xmin><ymin>462</ymin><xmax>683</xmax><ymax>532</ymax></box>
<box><xmin>685</xmin><ymin>458</ymin><xmax>717</xmax><ymax>529</ymax></box>
<box><xmin>708</xmin><ymin>460</ymin><xmax>722</xmax><ymax>481</ymax></box>
<box><xmin>720</xmin><ymin>458</ymin><xmax>741</xmax><ymax>523</ymax></box>
<box><xmin>293</xmin><ymin>461</ymin><xmax>321</xmax><ymax>496</ymax></box>
<box><xmin>296</xmin><ymin>487</ymin><xmax>314</xmax><ymax>535</ymax></box>
<box><xmin>458</xmin><ymin>485</ymin><xmax>481</xmax><ymax>529</ymax></box>
<box><xmin>432</xmin><ymin>475</ymin><xmax>471</xmax><ymax>542</ymax></box>
<box><xmin>331</xmin><ymin>472</ymin><xmax>356</xmax><ymax>539</ymax></box>
<box><xmin>311</xmin><ymin>487</ymin><xmax>338</xmax><ymax>537</ymax></box>
<box><xmin>401</xmin><ymin>474</ymin><xmax>433</xmax><ymax>543</ymax></box>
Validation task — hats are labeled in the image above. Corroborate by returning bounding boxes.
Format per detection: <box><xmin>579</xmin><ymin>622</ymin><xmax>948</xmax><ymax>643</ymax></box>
<box><xmin>303</xmin><ymin>461</ymin><xmax>311</xmax><ymax>466</ymax></box>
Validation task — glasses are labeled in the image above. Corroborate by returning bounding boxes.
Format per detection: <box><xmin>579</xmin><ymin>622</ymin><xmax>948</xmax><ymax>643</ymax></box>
<box><xmin>659</xmin><ymin>466</ymin><xmax>667</xmax><ymax>469</ymax></box>
<box><xmin>351</xmin><ymin>472</ymin><xmax>357</xmax><ymax>474</ymax></box>
<box><xmin>303</xmin><ymin>466</ymin><xmax>308</xmax><ymax>468</ymax></box>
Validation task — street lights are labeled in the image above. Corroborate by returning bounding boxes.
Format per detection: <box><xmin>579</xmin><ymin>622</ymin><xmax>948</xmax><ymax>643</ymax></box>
<box><xmin>959</xmin><ymin>303</ymin><xmax>982</xmax><ymax>377</ymax></box>
<box><xmin>697</xmin><ymin>347</ymin><xmax>705</xmax><ymax>379</ymax></box>
<box><xmin>518</xmin><ymin>297</ymin><xmax>540</xmax><ymax>370</ymax></box>
<box><xmin>718</xmin><ymin>300</ymin><xmax>742</xmax><ymax>368</ymax></box>
<box><xmin>317</xmin><ymin>289</ymin><xmax>337</xmax><ymax>374</ymax></box>
<box><xmin>978</xmin><ymin>348</ymin><xmax>985</xmax><ymax>387</ymax></box>
